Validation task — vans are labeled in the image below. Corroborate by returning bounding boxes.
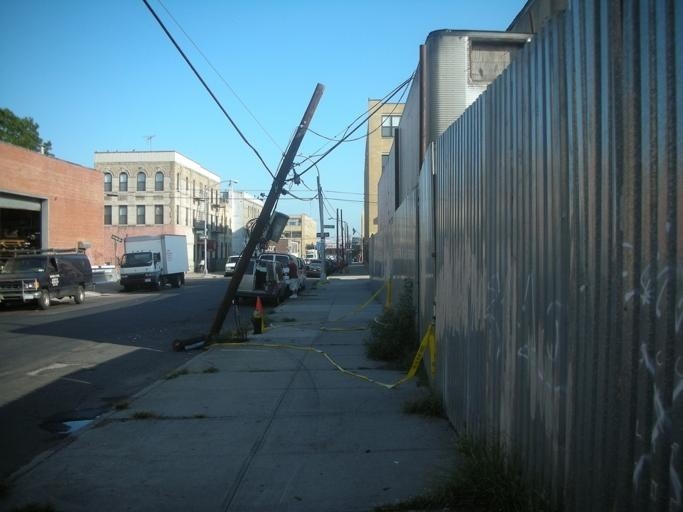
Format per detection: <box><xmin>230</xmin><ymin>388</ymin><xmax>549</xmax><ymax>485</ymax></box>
<box><xmin>0</xmin><ymin>252</ymin><xmax>92</xmax><ymax>312</ymax></box>
<box><xmin>236</xmin><ymin>259</ymin><xmax>290</xmax><ymax>304</ymax></box>
<box><xmin>258</xmin><ymin>252</ymin><xmax>306</xmax><ymax>294</ymax></box>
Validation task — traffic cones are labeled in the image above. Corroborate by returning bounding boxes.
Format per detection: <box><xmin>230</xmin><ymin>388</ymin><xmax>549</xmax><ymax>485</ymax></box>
<box><xmin>254</xmin><ymin>295</ymin><xmax>265</xmax><ymax>317</ymax></box>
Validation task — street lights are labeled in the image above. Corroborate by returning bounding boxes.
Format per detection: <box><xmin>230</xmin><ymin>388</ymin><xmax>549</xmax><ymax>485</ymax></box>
<box><xmin>280</xmin><ymin>152</ymin><xmax>324</xmax><ymax>279</ymax></box>
<box><xmin>204</xmin><ymin>180</ymin><xmax>239</xmax><ymax>274</ymax></box>
<box><xmin>329</xmin><ymin>217</ymin><xmax>350</xmax><ymax>260</ymax></box>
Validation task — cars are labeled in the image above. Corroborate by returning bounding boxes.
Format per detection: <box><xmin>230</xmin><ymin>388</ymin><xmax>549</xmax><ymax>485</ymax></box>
<box><xmin>225</xmin><ymin>253</ymin><xmax>242</xmax><ymax>274</ymax></box>
<box><xmin>303</xmin><ymin>249</ymin><xmax>337</xmax><ymax>279</ymax></box>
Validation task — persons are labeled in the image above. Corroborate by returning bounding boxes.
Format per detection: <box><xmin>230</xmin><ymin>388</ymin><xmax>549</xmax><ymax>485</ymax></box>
<box><xmin>281</xmin><ymin>260</ymin><xmax>300</xmax><ymax>298</ymax></box>
<box><xmin>198</xmin><ymin>258</ymin><xmax>205</xmax><ymax>270</ymax></box>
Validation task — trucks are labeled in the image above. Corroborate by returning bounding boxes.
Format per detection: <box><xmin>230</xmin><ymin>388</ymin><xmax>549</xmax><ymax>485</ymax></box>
<box><xmin>0</xmin><ymin>226</ymin><xmax>41</xmax><ymax>250</ymax></box>
<box><xmin>119</xmin><ymin>233</ymin><xmax>190</xmax><ymax>291</ymax></box>
